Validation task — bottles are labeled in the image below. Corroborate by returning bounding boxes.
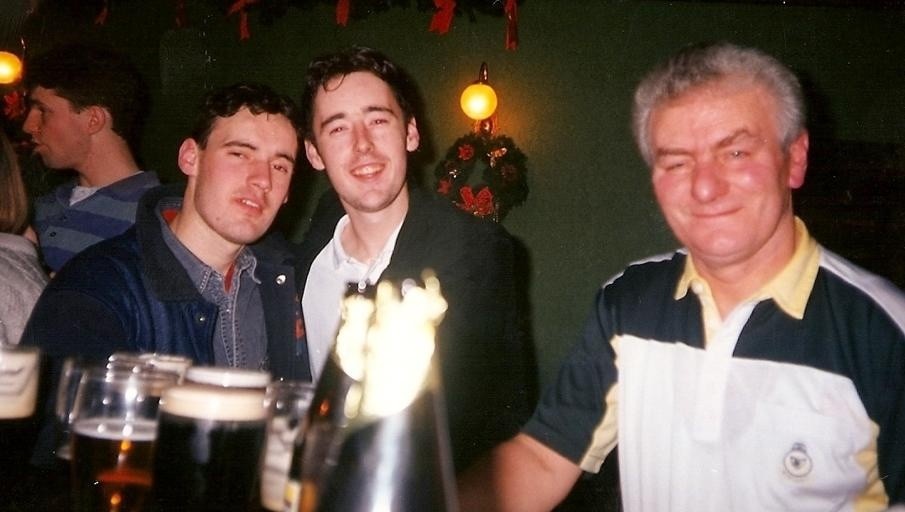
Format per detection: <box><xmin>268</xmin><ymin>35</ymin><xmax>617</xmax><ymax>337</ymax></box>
<box><xmin>283</xmin><ymin>270</ymin><xmax>448</xmax><ymax>512</ymax></box>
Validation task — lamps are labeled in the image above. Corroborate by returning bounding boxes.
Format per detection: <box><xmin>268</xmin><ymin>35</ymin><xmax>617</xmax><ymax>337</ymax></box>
<box><xmin>459</xmin><ymin>61</ymin><xmax>500</xmax><ymax>142</ymax></box>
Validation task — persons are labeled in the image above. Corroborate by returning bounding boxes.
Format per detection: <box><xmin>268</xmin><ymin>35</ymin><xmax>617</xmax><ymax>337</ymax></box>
<box><xmin>293</xmin><ymin>40</ymin><xmax>539</xmax><ymax>471</ymax></box>
<box><xmin>0</xmin><ymin>130</ymin><xmax>57</xmax><ymax>350</ymax></box>
<box><xmin>21</xmin><ymin>50</ymin><xmax>162</xmax><ymax>273</ymax></box>
<box><xmin>0</xmin><ymin>80</ymin><xmax>313</xmax><ymax>512</ymax></box>
<box><xmin>444</xmin><ymin>38</ymin><xmax>905</xmax><ymax>512</ymax></box>
